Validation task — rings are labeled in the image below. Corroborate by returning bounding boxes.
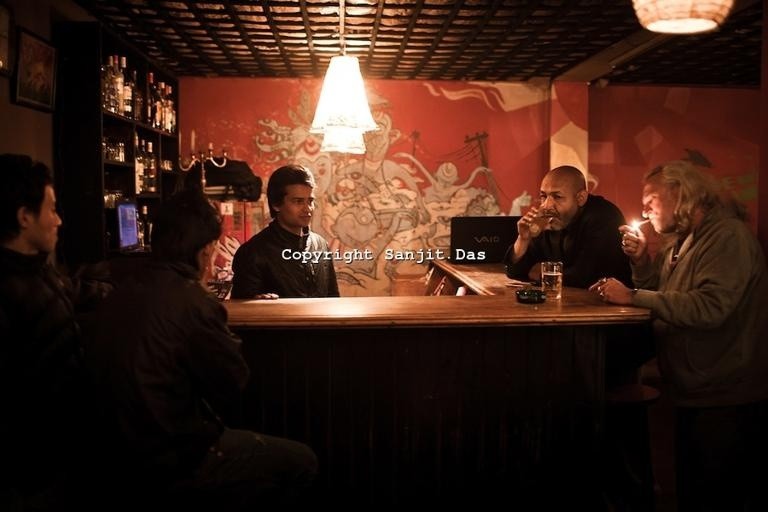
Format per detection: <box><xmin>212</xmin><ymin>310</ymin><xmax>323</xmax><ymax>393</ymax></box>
<box><xmin>598</xmin><ymin>291</ymin><xmax>603</xmax><ymax>297</ymax></box>
<box><xmin>622</xmin><ymin>239</ymin><xmax>625</xmax><ymax>247</ymax></box>
<box><xmin>599</xmin><ymin>276</ymin><xmax>607</xmax><ymax>282</ymax></box>
<box><xmin>596</xmin><ymin>285</ymin><xmax>602</xmax><ymax>293</ymax></box>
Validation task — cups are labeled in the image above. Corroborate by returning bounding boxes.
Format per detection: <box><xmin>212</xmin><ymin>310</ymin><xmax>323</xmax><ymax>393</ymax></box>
<box><xmin>540</xmin><ymin>261</ymin><xmax>565</xmax><ymax>303</ymax></box>
<box><xmin>526</xmin><ymin>212</ymin><xmax>553</xmax><ymax>237</ymax></box>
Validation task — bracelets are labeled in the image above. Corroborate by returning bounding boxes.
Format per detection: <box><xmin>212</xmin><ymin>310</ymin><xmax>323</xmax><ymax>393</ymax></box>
<box><xmin>627</xmin><ymin>285</ymin><xmax>641</xmax><ymax>306</ymax></box>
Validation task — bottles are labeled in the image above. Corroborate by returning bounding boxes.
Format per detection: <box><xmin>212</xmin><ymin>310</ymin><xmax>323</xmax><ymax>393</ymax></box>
<box><xmin>100</xmin><ymin>54</ymin><xmax>176</xmax><ymax>254</ymax></box>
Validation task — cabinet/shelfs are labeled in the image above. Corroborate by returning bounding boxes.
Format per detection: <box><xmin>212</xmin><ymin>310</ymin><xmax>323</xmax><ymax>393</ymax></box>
<box><xmin>54</xmin><ymin>28</ymin><xmax>181</xmax><ymax>267</ymax></box>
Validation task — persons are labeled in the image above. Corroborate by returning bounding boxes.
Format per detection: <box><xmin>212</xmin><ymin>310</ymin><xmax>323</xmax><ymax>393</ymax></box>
<box><xmin>0</xmin><ymin>153</ymin><xmax>121</xmax><ymax>512</ymax></box>
<box><xmin>230</xmin><ymin>167</ymin><xmax>340</xmax><ymax>300</ymax></box>
<box><xmin>582</xmin><ymin>160</ymin><xmax>767</xmax><ymax>512</ymax></box>
<box><xmin>90</xmin><ymin>191</ymin><xmax>319</xmax><ymax>512</ymax></box>
<box><xmin>499</xmin><ymin>164</ymin><xmax>627</xmax><ymax>295</ymax></box>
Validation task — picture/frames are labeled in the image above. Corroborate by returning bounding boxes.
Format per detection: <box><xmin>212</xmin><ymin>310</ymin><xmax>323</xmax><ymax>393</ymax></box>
<box><xmin>9</xmin><ymin>26</ymin><xmax>58</xmax><ymax>113</ymax></box>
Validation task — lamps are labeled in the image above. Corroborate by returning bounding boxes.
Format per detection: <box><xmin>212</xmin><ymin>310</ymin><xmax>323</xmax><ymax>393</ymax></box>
<box><xmin>309</xmin><ymin>1</ymin><xmax>380</xmax><ymax>134</ymax></box>
<box><xmin>630</xmin><ymin>0</ymin><xmax>735</xmax><ymax>36</ymax></box>
<box><xmin>318</xmin><ymin>129</ymin><xmax>367</xmax><ymax>156</ymax></box>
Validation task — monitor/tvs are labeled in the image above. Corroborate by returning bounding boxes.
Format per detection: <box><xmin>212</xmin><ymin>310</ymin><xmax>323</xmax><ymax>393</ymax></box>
<box><xmin>118</xmin><ymin>202</ymin><xmax>138</xmax><ymax>245</ymax></box>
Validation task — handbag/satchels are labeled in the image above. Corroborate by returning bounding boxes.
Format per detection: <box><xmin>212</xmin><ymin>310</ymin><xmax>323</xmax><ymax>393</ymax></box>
<box><xmin>192</xmin><ymin>158</ymin><xmax>262</xmax><ymax>201</ymax></box>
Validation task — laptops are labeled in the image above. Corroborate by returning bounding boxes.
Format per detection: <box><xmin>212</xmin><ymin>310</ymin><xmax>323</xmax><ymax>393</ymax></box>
<box><xmin>447</xmin><ymin>216</ymin><xmax>528</xmax><ymax>265</ymax></box>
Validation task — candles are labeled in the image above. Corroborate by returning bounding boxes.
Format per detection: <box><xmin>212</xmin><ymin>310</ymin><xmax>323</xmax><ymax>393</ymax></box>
<box><xmin>178</xmin><ymin>127</ymin><xmax>214</xmax><ymax>158</ymax></box>
